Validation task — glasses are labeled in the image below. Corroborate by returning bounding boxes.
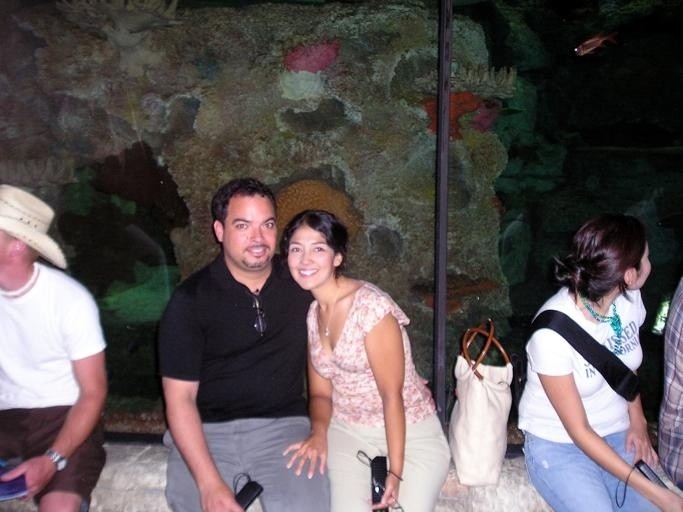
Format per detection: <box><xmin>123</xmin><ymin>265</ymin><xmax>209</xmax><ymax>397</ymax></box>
<box><xmin>372</xmin><ymin>478</ymin><xmax>404</xmax><ymax>512</ymax></box>
<box><xmin>252</xmin><ymin>289</ymin><xmax>267</xmax><ymax>336</ymax></box>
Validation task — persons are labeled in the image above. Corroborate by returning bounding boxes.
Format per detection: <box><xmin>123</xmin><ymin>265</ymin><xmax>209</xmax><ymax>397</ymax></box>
<box><xmin>157</xmin><ymin>179</ymin><xmax>332</xmax><ymax>512</ymax></box>
<box><xmin>280</xmin><ymin>209</ymin><xmax>451</xmax><ymax>512</ymax></box>
<box><xmin>657</xmin><ymin>276</ymin><xmax>682</xmax><ymax>488</ymax></box>
<box><xmin>517</xmin><ymin>214</ymin><xmax>683</xmax><ymax>511</ymax></box>
<box><xmin>0</xmin><ymin>183</ymin><xmax>109</xmax><ymax>512</ymax></box>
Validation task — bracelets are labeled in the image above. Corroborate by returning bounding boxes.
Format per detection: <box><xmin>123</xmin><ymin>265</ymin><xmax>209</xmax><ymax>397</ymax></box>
<box><xmin>387</xmin><ymin>470</ymin><xmax>403</xmax><ymax>482</ymax></box>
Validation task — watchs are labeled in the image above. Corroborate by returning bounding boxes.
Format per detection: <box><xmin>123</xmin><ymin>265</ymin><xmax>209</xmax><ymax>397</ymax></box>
<box><xmin>45</xmin><ymin>449</ymin><xmax>68</xmax><ymax>472</ymax></box>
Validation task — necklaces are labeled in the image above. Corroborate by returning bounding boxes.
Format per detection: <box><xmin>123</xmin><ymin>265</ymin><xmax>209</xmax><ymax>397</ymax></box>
<box><xmin>578</xmin><ymin>293</ymin><xmax>624</xmax><ymax>358</ymax></box>
<box><xmin>317</xmin><ymin>316</ymin><xmax>331</xmax><ymax>336</ymax></box>
<box><xmin>2</xmin><ymin>269</ymin><xmax>41</xmax><ymax>300</ymax></box>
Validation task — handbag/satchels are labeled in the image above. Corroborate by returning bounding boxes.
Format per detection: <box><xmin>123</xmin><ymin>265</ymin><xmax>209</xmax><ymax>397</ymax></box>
<box><xmin>448</xmin><ymin>318</ymin><xmax>513</xmax><ymax>486</ymax></box>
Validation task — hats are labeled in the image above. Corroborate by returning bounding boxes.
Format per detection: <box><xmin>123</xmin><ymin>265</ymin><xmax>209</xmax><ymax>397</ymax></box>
<box><xmin>0</xmin><ymin>184</ymin><xmax>67</xmax><ymax>270</ymax></box>
